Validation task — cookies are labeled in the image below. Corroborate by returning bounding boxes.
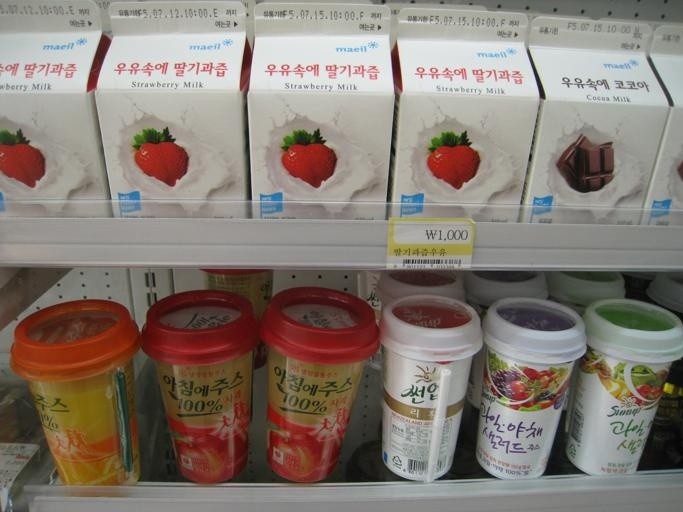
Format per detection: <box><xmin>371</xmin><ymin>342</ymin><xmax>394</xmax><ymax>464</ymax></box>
<box><xmin>557</xmin><ymin>133</ymin><xmax>615</xmax><ymax>192</ymax></box>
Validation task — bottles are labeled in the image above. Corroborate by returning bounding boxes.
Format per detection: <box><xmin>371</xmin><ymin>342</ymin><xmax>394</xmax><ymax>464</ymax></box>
<box><xmin>8</xmin><ymin>299</ymin><xmax>139</xmax><ymax>495</ymax></box>
<box><xmin>545</xmin><ymin>271</ymin><xmax>629</xmax><ymax>310</ymax></box>
<box><xmin>378</xmin><ymin>295</ymin><xmax>485</xmax><ymax>483</ymax></box>
<box><xmin>258</xmin><ymin>287</ymin><xmax>381</xmax><ymax>485</ymax></box>
<box><xmin>641</xmin><ymin>273</ymin><xmax>682</xmax><ymax>428</ymax></box>
<box><xmin>136</xmin><ymin>289</ymin><xmax>258</xmax><ymax>485</ymax></box>
<box><xmin>462</xmin><ymin>270</ymin><xmax>550</xmax><ymax>411</ymax></box>
<box><xmin>565</xmin><ymin>299</ymin><xmax>683</xmax><ymax>474</ymax></box>
<box><xmin>475</xmin><ymin>298</ymin><xmax>587</xmax><ymax>478</ymax></box>
<box><xmin>375</xmin><ymin>270</ymin><xmax>469</xmax><ymax>315</ymax></box>
<box><xmin>200</xmin><ymin>267</ymin><xmax>272</xmax><ymax>370</ymax></box>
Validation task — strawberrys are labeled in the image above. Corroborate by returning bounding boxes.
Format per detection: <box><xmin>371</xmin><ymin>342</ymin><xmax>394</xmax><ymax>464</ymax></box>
<box><xmin>0</xmin><ymin>128</ymin><xmax>45</xmax><ymax>189</ymax></box>
<box><xmin>280</xmin><ymin>128</ymin><xmax>337</xmax><ymax>188</ymax></box>
<box><xmin>131</xmin><ymin>127</ymin><xmax>189</xmax><ymax>188</ymax></box>
<box><xmin>426</xmin><ymin>129</ymin><xmax>481</xmax><ymax>190</ymax></box>
